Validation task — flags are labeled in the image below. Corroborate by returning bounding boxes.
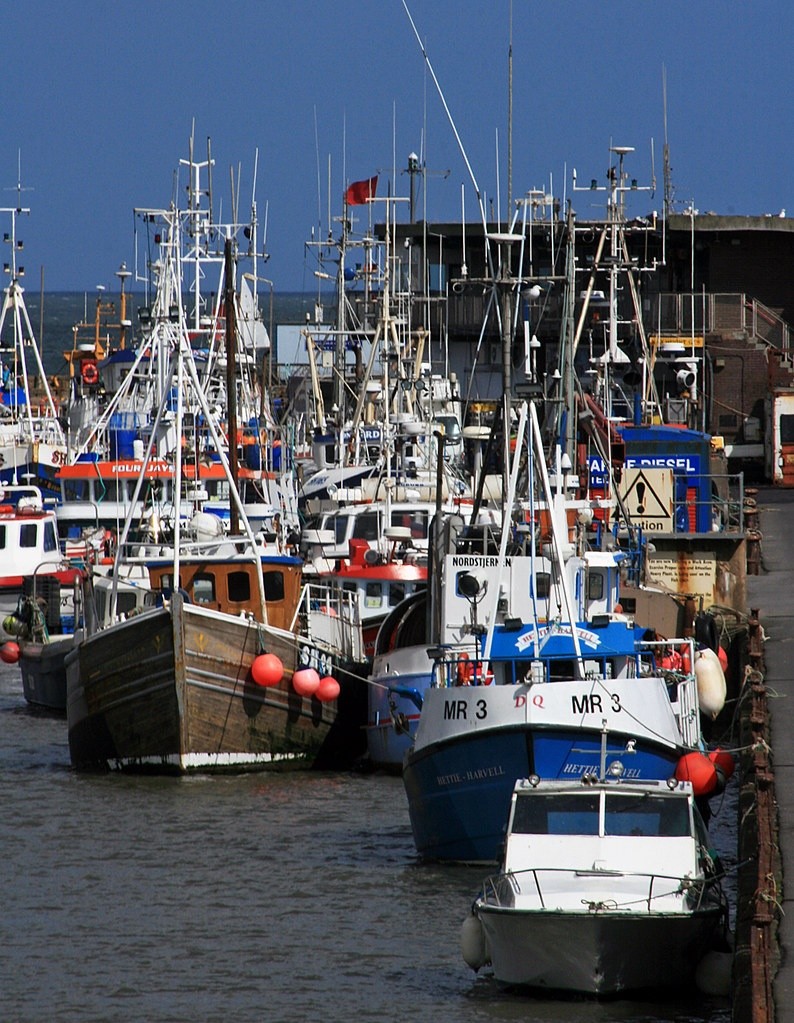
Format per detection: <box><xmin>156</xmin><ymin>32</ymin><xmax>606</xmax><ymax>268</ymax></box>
<box><xmin>348</xmin><ymin>175</ymin><xmax>378</xmax><ymax>206</ymax></box>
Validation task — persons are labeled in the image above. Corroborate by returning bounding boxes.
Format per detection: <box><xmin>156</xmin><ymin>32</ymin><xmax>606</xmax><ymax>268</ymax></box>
<box><xmin>4</xmin><ymin>364</ymin><xmax>10</xmax><ymax>382</ymax></box>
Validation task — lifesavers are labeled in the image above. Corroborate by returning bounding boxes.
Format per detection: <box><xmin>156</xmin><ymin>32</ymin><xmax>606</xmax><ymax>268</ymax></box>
<box><xmin>615</xmin><ymin>604</ymin><xmax>623</xmax><ymax>620</ymax></box>
<box><xmin>0</xmin><ymin>506</ymin><xmax>13</xmax><ymax>513</ymax></box>
<box><xmin>83</xmin><ymin>364</ymin><xmax>97</xmax><ymax>384</ymax></box>
<box><xmin>680</xmin><ymin>644</ymin><xmax>690</xmax><ymax>675</ymax></box>
<box><xmin>90</xmin><ymin>557</ymin><xmax>114</xmax><ymax>564</ymax></box>
<box><xmin>40</xmin><ymin>396</ymin><xmax>58</xmax><ymax>416</ymax></box>
<box><xmin>445</xmin><ymin>498</ymin><xmax>474</xmax><ymax>505</ymax></box>
<box><xmin>458</xmin><ymin>653</ymin><xmax>469</xmax><ymax>685</ymax></box>
<box><xmin>320</xmin><ymin>605</ymin><xmax>336</xmax><ymax>618</ymax></box>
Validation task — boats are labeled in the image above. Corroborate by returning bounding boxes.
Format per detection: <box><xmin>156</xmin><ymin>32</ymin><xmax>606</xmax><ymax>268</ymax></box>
<box><xmin>460</xmin><ymin>712</ymin><xmax>738</xmax><ymax>1000</ymax></box>
<box><xmin>0</xmin><ymin>0</ymin><xmax>739</xmax><ymax>868</ymax></box>
